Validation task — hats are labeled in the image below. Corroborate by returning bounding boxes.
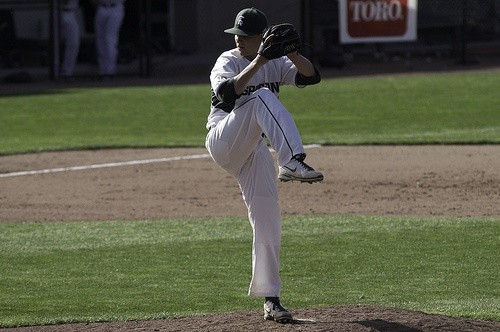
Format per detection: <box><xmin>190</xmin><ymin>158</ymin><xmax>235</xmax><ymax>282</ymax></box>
<box><xmin>223</xmin><ymin>7</ymin><xmax>270</xmax><ymax>37</ymax></box>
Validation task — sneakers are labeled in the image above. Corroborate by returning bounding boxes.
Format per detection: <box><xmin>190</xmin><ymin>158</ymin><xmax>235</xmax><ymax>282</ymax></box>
<box><xmin>278</xmin><ymin>154</ymin><xmax>324</xmax><ymax>183</ymax></box>
<box><xmin>264</xmin><ymin>300</ymin><xmax>293</xmax><ymax>323</ymax></box>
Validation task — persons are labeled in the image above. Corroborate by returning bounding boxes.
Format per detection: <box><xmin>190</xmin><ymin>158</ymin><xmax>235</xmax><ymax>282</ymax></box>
<box><xmin>57</xmin><ymin>0</ymin><xmax>83</xmax><ymax>80</ymax></box>
<box><xmin>94</xmin><ymin>0</ymin><xmax>125</xmax><ymax>81</ymax></box>
<box><xmin>206</xmin><ymin>7</ymin><xmax>324</xmax><ymax>324</ymax></box>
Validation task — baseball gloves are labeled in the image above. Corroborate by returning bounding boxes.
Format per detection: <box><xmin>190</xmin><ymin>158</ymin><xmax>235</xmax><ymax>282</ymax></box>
<box><xmin>257</xmin><ymin>23</ymin><xmax>303</xmax><ymax>60</ymax></box>
